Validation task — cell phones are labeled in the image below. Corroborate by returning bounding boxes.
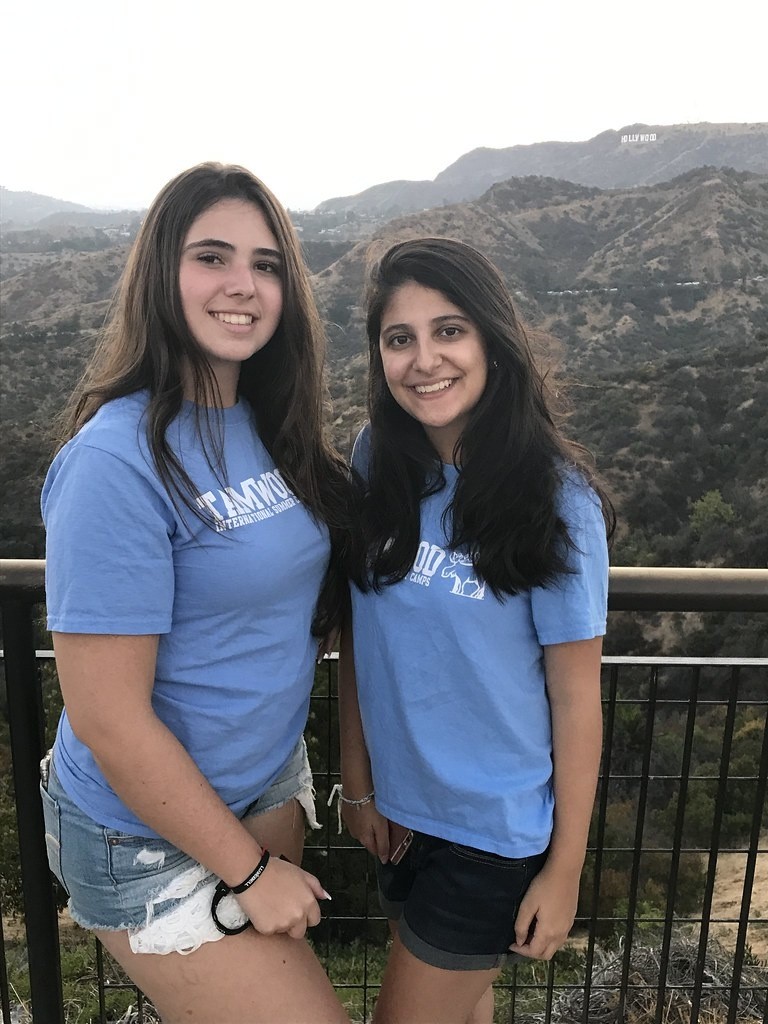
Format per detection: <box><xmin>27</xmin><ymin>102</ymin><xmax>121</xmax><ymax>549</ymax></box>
<box><xmin>387</xmin><ymin>819</ymin><xmax>416</xmax><ymax>865</ymax></box>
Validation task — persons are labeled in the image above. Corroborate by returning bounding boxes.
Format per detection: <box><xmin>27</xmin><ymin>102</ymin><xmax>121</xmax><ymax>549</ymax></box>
<box><xmin>35</xmin><ymin>163</ymin><xmax>354</xmax><ymax>1024</ymax></box>
<box><xmin>333</xmin><ymin>236</ymin><xmax>611</xmax><ymax>1024</ymax></box>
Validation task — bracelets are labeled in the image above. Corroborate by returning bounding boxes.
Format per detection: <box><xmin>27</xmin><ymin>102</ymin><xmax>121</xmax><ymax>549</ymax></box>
<box><xmin>231</xmin><ymin>847</ymin><xmax>271</xmax><ymax>894</ymax></box>
<box><xmin>340</xmin><ymin>790</ymin><xmax>375</xmax><ymax>811</ymax></box>
